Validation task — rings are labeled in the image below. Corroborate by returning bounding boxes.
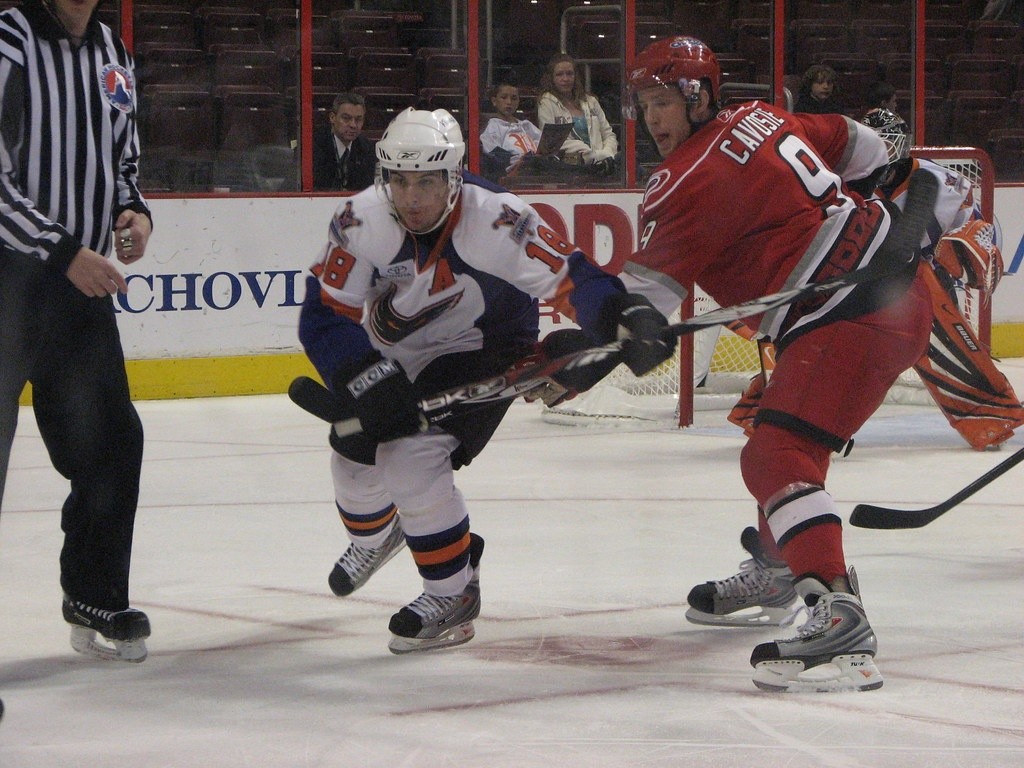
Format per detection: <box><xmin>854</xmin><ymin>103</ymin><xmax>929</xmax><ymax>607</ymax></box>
<box><xmin>121</xmin><ymin>228</ymin><xmax>132</xmax><ymax>253</ymax></box>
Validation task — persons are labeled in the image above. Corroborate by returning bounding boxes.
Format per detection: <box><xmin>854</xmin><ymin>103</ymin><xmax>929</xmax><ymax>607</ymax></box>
<box><xmin>0</xmin><ymin>0</ymin><xmax>155</xmax><ymax>722</ymax></box>
<box><xmin>279</xmin><ymin>92</ymin><xmax>373</xmax><ymax>193</ymax></box>
<box><xmin>791</xmin><ymin>65</ymin><xmax>838</xmax><ymax>113</ymax></box>
<box><xmin>479</xmin><ymin>75</ymin><xmax>604</xmax><ymax>183</ymax></box>
<box><xmin>300</xmin><ymin>104</ymin><xmax>680</xmax><ymax>655</ymax></box>
<box><xmin>504</xmin><ymin>35</ymin><xmax>933</xmax><ymax>693</ymax></box>
<box><xmin>538</xmin><ymin>53</ymin><xmax>647</xmax><ymax>182</ymax></box>
<box><xmin>727</xmin><ymin>108</ymin><xmax>1005</xmax><ymax>437</ymax></box>
<box><xmin>862</xmin><ymin>81</ymin><xmax>897</xmax><ymax>123</ymax></box>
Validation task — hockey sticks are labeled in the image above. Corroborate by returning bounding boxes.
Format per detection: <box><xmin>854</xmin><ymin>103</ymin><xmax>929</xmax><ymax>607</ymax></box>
<box><xmin>848</xmin><ymin>448</ymin><xmax>1024</xmax><ymax>531</ymax></box>
<box><xmin>333</xmin><ymin>167</ymin><xmax>940</xmax><ymax>440</ymax></box>
<box><xmin>286</xmin><ymin>374</ymin><xmax>550</xmax><ymax>422</ymax></box>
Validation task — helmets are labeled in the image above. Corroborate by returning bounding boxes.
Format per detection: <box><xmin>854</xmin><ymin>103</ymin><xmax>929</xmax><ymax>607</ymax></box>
<box><xmin>376</xmin><ymin>106</ymin><xmax>465</xmax><ymax>194</ymax></box>
<box><xmin>862</xmin><ymin>108</ymin><xmax>910</xmax><ymax>139</ymax></box>
<box><xmin>633</xmin><ymin>36</ymin><xmax>720</xmax><ymax>102</ymax></box>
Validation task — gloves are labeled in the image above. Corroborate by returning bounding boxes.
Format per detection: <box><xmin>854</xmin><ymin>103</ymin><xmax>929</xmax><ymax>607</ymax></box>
<box><xmin>540</xmin><ymin>327</ymin><xmax>609</xmax><ymax>394</ymax></box>
<box><xmin>347</xmin><ymin>356</ymin><xmax>420</xmax><ymax>443</ymax></box>
<box><xmin>599</xmin><ymin>296</ymin><xmax>677</xmax><ymax>375</ymax></box>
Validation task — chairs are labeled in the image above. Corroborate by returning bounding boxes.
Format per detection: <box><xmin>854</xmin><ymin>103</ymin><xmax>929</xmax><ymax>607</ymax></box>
<box><xmin>99</xmin><ymin>0</ymin><xmax>1024</xmax><ymax>193</ymax></box>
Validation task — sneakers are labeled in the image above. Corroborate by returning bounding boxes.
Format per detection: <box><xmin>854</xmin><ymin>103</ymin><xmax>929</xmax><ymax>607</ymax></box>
<box><xmin>62</xmin><ymin>592</ymin><xmax>151</xmax><ymax>663</ymax></box>
<box><xmin>389</xmin><ymin>533</ymin><xmax>484</xmax><ymax>653</ymax></box>
<box><xmin>685</xmin><ymin>526</ymin><xmax>800</xmax><ymax>627</ymax></box>
<box><xmin>749</xmin><ymin>563</ymin><xmax>884</xmax><ymax>692</ymax></box>
<box><xmin>328</xmin><ymin>512</ymin><xmax>409</xmax><ymax>596</ymax></box>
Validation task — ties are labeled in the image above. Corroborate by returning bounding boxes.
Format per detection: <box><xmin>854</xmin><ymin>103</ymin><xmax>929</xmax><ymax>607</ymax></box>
<box><xmin>341</xmin><ymin>148</ymin><xmax>348</xmax><ymax>180</ymax></box>
